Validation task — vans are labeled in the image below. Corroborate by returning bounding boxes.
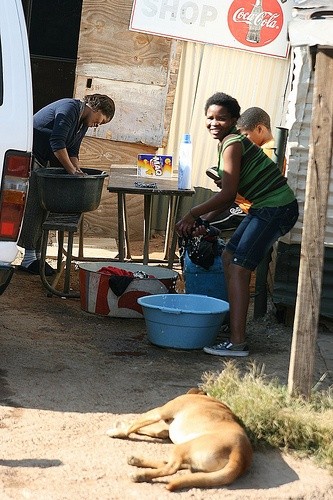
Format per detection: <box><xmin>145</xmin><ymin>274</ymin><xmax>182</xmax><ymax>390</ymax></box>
<box><xmin>0</xmin><ymin>0</ymin><xmax>34</xmax><ymax>296</ymax></box>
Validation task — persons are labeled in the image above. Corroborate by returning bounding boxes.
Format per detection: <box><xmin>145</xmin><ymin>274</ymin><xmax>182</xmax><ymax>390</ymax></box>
<box><xmin>17</xmin><ymin>94</ymin><xmax>115</xmax><ymax>274</ymax></box>
<box><xmin>209</xmin><ymin>107</ymin><xmax>287</xmax><ymax>230</ymax></box>
<box><xmin>175</xmin><ymin>92</ymin><xmax>299</xmax><ymax>357</ymax></box>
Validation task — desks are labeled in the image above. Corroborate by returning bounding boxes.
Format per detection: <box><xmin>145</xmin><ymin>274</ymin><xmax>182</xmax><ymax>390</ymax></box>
<box><xmin>106</xmin><ymin>164</ymin><xmax>195</xmax><ymax>270</ymax></box>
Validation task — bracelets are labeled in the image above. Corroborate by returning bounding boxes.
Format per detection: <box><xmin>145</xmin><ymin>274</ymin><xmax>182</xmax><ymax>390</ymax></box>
<box><xmin>190</xmin><ymin>209</ymin><xmax>197</xmax><ymax>219</ymax></box>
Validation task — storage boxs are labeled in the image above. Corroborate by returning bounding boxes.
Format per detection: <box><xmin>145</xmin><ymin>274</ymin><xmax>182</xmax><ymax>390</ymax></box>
<box><xmin>137</xmin><ymin>154</ymin><xmax>173</xmax><ymax>180</ymax></box>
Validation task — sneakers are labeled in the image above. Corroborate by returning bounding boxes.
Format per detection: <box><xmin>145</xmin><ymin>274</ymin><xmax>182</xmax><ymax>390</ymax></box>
<box><xmin>215</xmin><ymin>324</ymin><xmax>231</xmax><ymax>340</ymax></box>
<box><xmin>204</xmin><ymin>340</ymin><xmax>249</xmax><ymax>357</ymax></box>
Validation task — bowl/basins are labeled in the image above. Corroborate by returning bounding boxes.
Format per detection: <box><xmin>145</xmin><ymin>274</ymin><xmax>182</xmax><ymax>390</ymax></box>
<box><xmin>137</xmin><ymin>294</ymin><xmax>230</xmax><ymax>350</ymax></box>
<box><xmin>33</xmin><ymin>166</ymin><xmax>110</xmax><ymax>213</ymax></box>
<box><xmin>78</xmin><ymin>262</ymin><xmax>178</xmax><ymax>320</ymax></box>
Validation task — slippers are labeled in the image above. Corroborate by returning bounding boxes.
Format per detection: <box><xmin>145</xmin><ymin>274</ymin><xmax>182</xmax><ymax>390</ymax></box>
<box><xmin>19</xmin><ymin>260</ymin><xmax>56</xmax><ymax>276</ymax></box>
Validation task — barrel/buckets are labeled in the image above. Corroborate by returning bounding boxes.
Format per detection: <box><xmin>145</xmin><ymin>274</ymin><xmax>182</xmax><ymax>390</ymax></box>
<box><xmin>185</xmin><ymin>239</ymin><xmax>228</xmax><ymax>302</ymax></box>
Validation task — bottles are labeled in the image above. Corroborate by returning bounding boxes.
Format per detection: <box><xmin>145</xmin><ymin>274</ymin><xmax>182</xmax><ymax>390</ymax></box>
<box><xmin>177</xmin><ymin>133</ymin><xmax>193</xmax><ymax>190</ymax></box>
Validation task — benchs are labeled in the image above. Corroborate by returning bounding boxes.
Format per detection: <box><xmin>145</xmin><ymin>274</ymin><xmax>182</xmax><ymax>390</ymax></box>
<box><xmin>38</xmin><ymin>211</ymin><xmax>84</xmax><ymax>297</ymax></box>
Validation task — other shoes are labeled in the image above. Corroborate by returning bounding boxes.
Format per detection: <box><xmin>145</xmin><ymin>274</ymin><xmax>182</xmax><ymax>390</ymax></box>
<box><xmin>206</xmin><ymin>167</ymin><xmax>221</xmax><ymax>180</ymax></box>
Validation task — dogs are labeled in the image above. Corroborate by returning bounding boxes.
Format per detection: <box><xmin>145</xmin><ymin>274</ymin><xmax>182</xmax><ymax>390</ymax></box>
<box><xmin>104</xmin><ymin>386</ymin><xmax>254</xmax><ymax>492</ymax></box>
